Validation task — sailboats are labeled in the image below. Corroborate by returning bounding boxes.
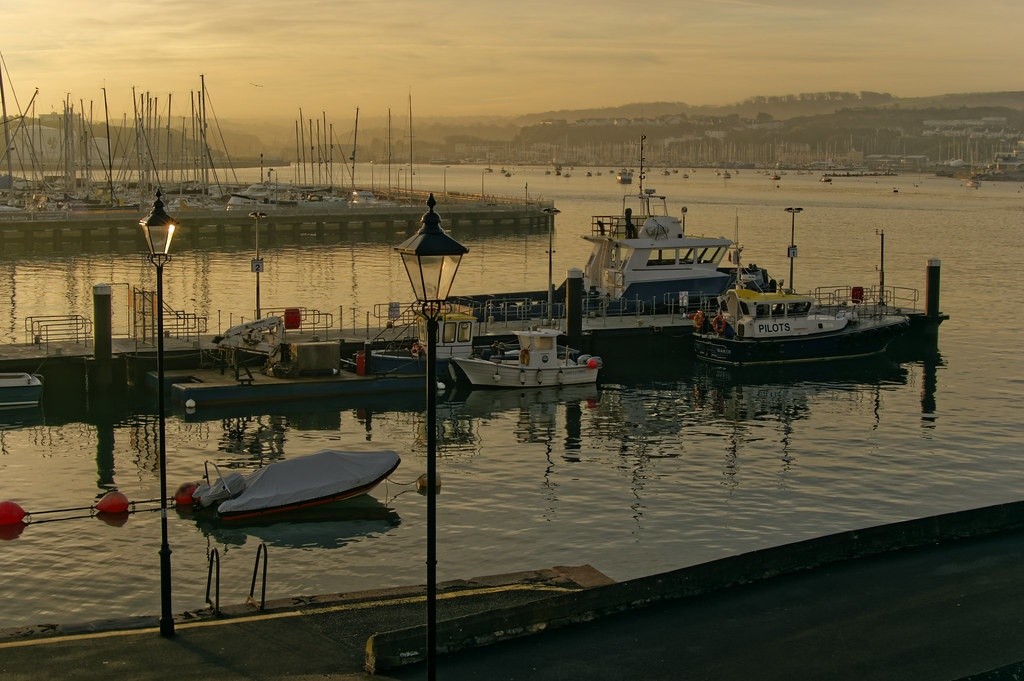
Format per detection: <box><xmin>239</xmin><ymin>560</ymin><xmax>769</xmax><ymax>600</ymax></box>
<box><xmin>0</xmin><ymin>52</ymin><xmax>424</xmax><ymax>213</ymax></box>
<box><xmin>450</xmin><ymin>129</ymin><xmax>1024</xmax><ymax>188</ymax></box>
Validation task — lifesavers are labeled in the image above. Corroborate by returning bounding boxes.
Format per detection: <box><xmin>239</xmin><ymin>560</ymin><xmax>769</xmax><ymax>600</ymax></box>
<box><xmin>693</xmin><ymin>310</ymin><xmax>703</xmax><ymax>328</ymax></box>
<box><xmin>712</xmin><ymin>315</ymin><xmax>726</xmax><ymax>333</ymax></box>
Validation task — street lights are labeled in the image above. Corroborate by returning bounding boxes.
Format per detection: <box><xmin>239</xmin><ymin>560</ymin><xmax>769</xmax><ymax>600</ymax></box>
<box><xmin>394</xmin><ymin>193</ymin><xmax>471</xmax><ymax>681</ymax></box>
<box><xmin>783</xmin><ymin>207</ymin><xmax>801</xmax><ymax>292</ymax></box>
<box><xmin>137</xmin><ymin>188</ymin><xmax>181</xmax><ymax>638</ymax></box>
<box><xmin>541</xmin><ymin>207</ymin><xmax>561</xmax><ymax>323</ymax></box>
<box><xmin>249</xmin><ymin>212</ymin><xmax>268</xmax><ymax>319</ymax></box>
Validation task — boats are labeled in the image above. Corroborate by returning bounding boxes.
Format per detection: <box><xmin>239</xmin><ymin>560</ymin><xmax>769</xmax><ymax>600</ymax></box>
<box><xmin>0</xmin><ymin>372</ymin><xmax>43</xmax><ymax>412</ymax></box>
<box><xmin>193</xmin><ymin>448</ymin><xmax>401</xmax><ymax>521</ymax></box>
<box><xmin>444</xmin><ymin>133</ymin><xmax>787</xmax><ymax>321</ymax></box>
<box><xmin>691</xmin><ymin>288</ymin><xmax>909</xmax><ymax>367</ymax></box>
<box><xmin>451</xmin><ymin>328</ymin><xmax>604</xmax><ymax>388</ymax></box>
<box><xmin>354</xmin><ymin>315</ymin><xmax>585</xmax><ymax>389</ymax></box>
